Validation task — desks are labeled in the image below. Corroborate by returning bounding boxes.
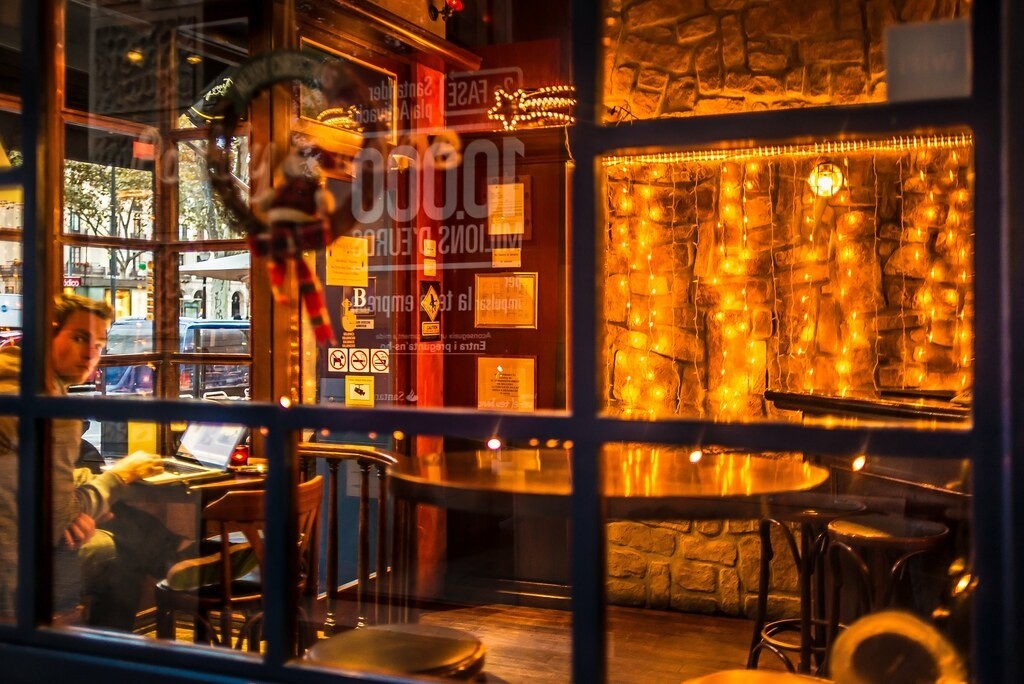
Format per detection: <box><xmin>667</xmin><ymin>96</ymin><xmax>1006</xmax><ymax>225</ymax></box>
<box><xmin>385</xmin><ymin>450</ymin><xmax>829</xmax><ymax>519</ymax></box>
<box><xmin>125</xmin><ymin>455</ymin><xmax>271</xmax><ymax>646</ymax></box>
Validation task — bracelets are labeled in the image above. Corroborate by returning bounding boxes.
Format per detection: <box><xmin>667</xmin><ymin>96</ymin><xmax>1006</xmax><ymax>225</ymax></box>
<box><xmin>112</xmin><ymin>471</ymin><xmax>127</xmax><ymax>487</ymax></box>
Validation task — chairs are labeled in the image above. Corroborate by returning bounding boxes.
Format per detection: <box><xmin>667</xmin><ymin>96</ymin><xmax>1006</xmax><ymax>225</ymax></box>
<box><xmin>155</xmin><ymin>475</ymin><xmax>322</xmax><ymax>660</ymax></box>
<box><xmin>209</xmin><ymin>333</ymin><xmax>249</xmax><ymax>355</ymax></box>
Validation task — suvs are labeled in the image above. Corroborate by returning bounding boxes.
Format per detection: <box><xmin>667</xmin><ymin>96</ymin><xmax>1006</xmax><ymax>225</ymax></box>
<box><xmin>98</xmin><ymin>320</ymin><xmax>249</xmax><ymax>399</ymax></box>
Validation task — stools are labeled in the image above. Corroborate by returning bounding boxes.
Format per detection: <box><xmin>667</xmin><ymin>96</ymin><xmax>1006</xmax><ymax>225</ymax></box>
<box><xmin>300</xmin><ymin>622</ymin><xmax>488</xmax><ymax>682</ymax></box>
<box><xmin>746</xmin><ymin>493</ymin><xmax>950</xmax><ymax>677</ymax></box>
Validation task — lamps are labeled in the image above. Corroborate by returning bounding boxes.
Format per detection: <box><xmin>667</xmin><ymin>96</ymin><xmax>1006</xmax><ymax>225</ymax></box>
<box><xmin>429</xmin><ymin>0</ymin><xmax>464</xmax><ymax>21</ymax></box>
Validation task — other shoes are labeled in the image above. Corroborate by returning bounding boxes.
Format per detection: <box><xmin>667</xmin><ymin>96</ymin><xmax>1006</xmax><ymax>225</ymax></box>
<box><xmin>166</xmin><ymin>538</ymin><xmax>259</xmax><ymax>590</ymax></box>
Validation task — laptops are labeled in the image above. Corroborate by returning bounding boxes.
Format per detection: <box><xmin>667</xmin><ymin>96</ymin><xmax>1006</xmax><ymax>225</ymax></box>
<box><xmin>140</xmin><ymin>425</ymin><xmax>247</xmax><ymax>485</ymax></box>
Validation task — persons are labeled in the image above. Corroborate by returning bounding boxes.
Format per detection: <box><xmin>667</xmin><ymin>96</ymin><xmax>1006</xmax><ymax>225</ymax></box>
<box><xmin>0</xmin><ymin>294</ymin><xmax>259</xmax><ymax>635</ymax></box>
